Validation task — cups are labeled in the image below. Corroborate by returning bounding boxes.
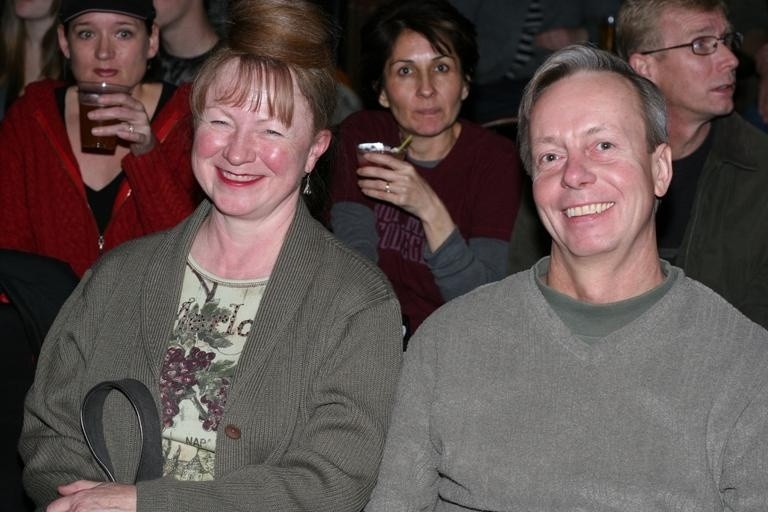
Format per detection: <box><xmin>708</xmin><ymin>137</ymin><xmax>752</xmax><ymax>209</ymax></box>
<box><xmin>76</xmin><ymin>80</ymin><xmax>131</xmax><ymax>155</ymax></box>
<box><xmin>355</xmin><ymin>143</ymin><xmax>407</xmax><ymax>188</ymax></box>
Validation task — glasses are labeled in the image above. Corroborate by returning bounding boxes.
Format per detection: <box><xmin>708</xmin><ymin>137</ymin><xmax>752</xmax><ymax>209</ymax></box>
<box><xmin>641</xmin><ymin>30</ymin><xmax>744</xmax><ymax>55</ymax></box>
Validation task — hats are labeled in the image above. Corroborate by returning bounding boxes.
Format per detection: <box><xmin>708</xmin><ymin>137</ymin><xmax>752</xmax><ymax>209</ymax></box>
<box><xmin>59</xmin><ymin>0</ymin><xmax>157</xmax><ymax>22</ymax></box>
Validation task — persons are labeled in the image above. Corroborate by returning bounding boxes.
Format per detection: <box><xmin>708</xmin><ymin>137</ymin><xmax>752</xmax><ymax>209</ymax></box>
<box><xmin>21</xmin><ymin>1</ymin><xmax>404</xmax><ymax>510</ymax></box>
<box><xmin>360</xmin><ymin>45</ymin><xmax>768</xmax><ymax>512</ymax></box>
<box><xmin>1</xmin><ymin>1</ymin><xmax>767</xmax><ymax>345</ymax></box>
